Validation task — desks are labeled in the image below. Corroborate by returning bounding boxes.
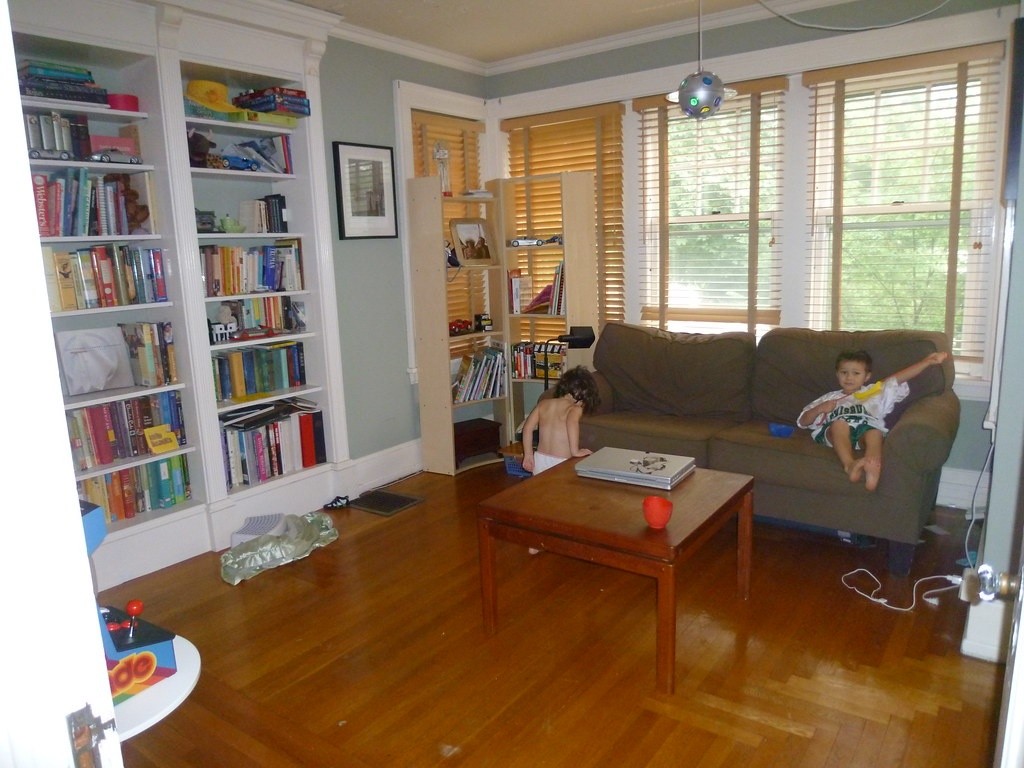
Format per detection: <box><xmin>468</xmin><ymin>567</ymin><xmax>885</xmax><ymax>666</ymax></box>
<box><xmin>477</xmin><ymin>452</ymin><xmax>756</xmax><ymax>694</ymax></box>
<box><xmin>114</xmin><ymin>633</ymin><xmax>202</xmax><ymax>742</ymax></box>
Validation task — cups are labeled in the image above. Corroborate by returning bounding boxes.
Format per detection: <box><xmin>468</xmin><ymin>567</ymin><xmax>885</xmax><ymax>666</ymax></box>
<box><xmin>221</xmin><ymin>213</ymin><xmax>239</xmax><ymax>226</ymax></box>
<box><xmin>643</xmin><ymin>496</ymin><xmax>673</xmax><ymax>528</ymax></box>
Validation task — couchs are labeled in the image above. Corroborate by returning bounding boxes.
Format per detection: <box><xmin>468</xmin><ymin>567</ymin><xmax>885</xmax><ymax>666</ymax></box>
<box><xmin>539</xmin><ymin>319</ymin><xmax>963</xmax><ymax>579</ymax></box>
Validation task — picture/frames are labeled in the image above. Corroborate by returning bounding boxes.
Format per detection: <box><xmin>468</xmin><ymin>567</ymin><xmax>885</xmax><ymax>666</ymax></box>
<box><xmin>333</xmin><ymin>141</ymin><xmax>399</xmax><ymax>239</ymax></box>
<box><xmin>448</xmin><ymin>217</ymin><xmax>498</xmax><ymax>267</ymax></box>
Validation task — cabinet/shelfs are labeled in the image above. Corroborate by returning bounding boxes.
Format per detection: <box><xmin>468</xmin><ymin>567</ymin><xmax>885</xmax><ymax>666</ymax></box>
<box><xmin>11</xmin><ymin>19</ymin><xmax>335</xmax><ymax>540</ymax></box>
<box><xmin>445</xmin><ymin>195</ymin><xmax>513</xmax><ymax>472</ymax></box>
<box><xmin>488</xmin><ymin>172</ymin><xmax>597</xmax><ymax>384</ymax></box>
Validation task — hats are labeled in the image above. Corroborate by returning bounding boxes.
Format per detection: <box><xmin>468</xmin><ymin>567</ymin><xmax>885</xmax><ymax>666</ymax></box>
<box><xmin>187</xmin><ymin>132</ymin><xmax>216</xmax><ymax>167</ymax></box>
<box><xmin>465</xmin><ymin>238</ymin><xmax>475</xmax><ymax>245</ymax></box>
<box><xmin>187</xmin><ymin>79</ymin><xmax>244</xmax><ymax>113</ymax></box>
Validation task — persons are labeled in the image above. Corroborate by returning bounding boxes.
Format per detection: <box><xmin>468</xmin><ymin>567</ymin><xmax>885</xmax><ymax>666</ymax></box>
<box><xmin>796</xmin><ymin>350</ymin><xmax>947</xmax><ymax>490</ymax></box>
<box><xmin>478</xmin><ymin>237</ymin><xmax>490</xmax><ymax>259</ymax></box>
<box><xmin>522</xmin><ymin>367</ymin><xmax>601</xmax><ymax>555</ymax></box>
<box><xmin>463</xmin><ymin>240</ymin><xmax>478</xmax><ymax>260</ymax></box>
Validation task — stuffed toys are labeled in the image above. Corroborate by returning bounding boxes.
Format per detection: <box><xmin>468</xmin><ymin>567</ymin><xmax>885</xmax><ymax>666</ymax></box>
<box><xmin>104</xmin><ymin>175</ymin><xmax>148</xmax><ymax>230</ymax></box>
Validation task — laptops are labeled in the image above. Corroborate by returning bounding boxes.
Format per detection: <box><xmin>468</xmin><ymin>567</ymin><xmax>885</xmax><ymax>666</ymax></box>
<box><xmin>575</xmin><ymin>447</ymin><xmax>696</xmax><ymax>490</ymax></box>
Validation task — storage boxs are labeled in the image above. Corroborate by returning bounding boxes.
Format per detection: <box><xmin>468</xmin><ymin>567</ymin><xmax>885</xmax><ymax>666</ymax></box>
<box><xmin>454</xmin><ymin>418</ymin><xmax>503</xmax><ymax>467</ymax></box>
<box><xmin>56</xmin><ymin>326</ymin><xmax>134</xmax><ymax>396</ymax></box>
<box><xmin>535</xmin><ymin>352</ymin><xmax>562</xmax><ymax>379</ymax></box>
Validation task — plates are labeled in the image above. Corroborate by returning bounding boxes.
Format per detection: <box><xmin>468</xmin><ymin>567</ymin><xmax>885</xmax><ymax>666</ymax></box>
<box><xmin>219</xmin><ymin>225</ymin><xmax>246</xmax><ymax>233</ymax></box>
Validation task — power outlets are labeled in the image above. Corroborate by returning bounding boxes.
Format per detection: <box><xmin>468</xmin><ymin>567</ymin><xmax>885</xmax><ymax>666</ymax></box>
<box><xmin>958</xmin><ymin>567</ymin><xmax>977</xmax><ymax>603</ymax></box>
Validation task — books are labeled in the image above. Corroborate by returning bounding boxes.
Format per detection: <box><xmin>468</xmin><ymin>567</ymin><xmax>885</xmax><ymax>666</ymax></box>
<box><xmin>513</xmin><ymin>340</ymin><xmax>566</xmax><ymax>379</ymax></box>
<box><xmin>509</xmin><ymin>260</ymin><xmax>565</xmax><ymax>315</ymax></box>
<box><xmin>15</xmin><ymin>61</ymin><xmax>193</xmax><ymax>523</ymax></box>
<box><xmin>226</xmin><ymin>88</ymin><xmax>311</xmax><ymax>129</ymax></box>
<box><xmin>199</xmin><ymin>193</ymin><xmax>327</xmax><ymax>498</ymax></box>
<box><xmin>222</xmin><ymin>139</ymin><xmax>292</xmax><ymax>174</ymax></box>
<box><xmin>453</xmin><ymin>339</ymin><xmax>507</xmax><ymax>404</ymax></box>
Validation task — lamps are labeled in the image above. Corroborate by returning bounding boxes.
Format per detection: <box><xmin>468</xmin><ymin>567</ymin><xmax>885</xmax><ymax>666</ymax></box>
<box><xmin>665</xmin><ymin>0</ymin><xmax>736</xmax><ymax>119</ymax></box>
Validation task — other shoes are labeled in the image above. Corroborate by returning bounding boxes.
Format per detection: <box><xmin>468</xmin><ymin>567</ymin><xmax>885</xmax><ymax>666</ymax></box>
<box><xmin>529</xmin><ymin>548</ymin><xmax>539</xmax><ymax>555</ymax></box>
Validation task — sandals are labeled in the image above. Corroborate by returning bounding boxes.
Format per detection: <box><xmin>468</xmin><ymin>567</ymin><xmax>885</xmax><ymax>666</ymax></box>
<box><xmin>324</xmin><ymin>496</ymin><xmax>350</xmax><ymax>510</ymax></box>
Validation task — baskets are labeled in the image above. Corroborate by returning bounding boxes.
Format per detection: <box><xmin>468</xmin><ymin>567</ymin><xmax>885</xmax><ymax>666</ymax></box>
<box><xmin>504</xmin><ymin>457</ymin><xmax>532</xmax><ymax>477</ymax></box>
